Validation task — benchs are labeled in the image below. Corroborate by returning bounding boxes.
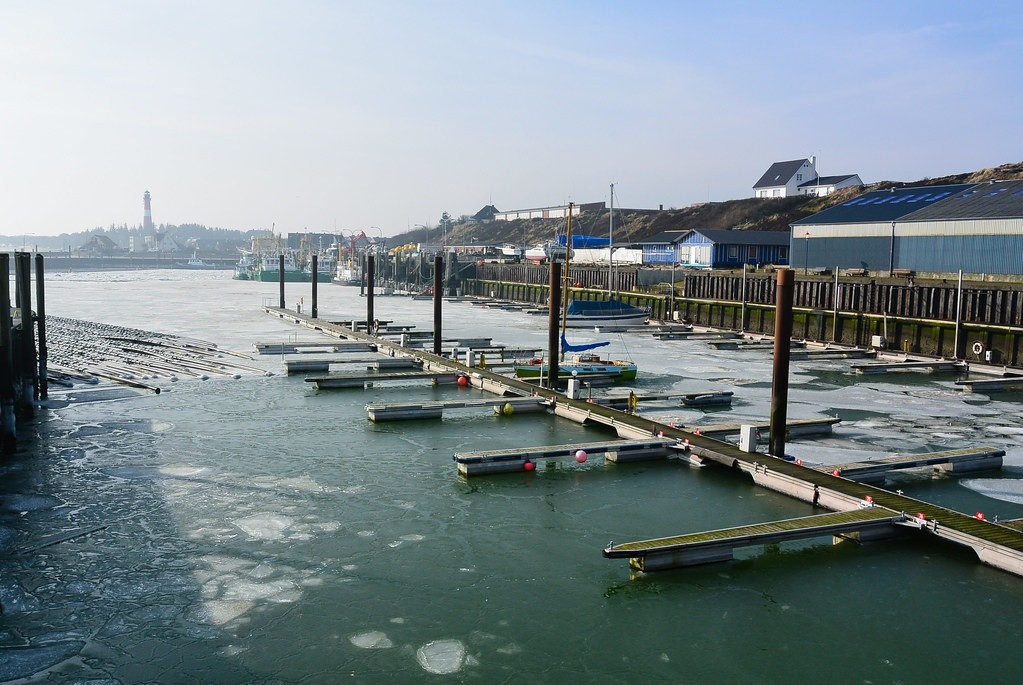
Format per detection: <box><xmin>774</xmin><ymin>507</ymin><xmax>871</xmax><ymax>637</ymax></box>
<box><xmin>846</xmin><ymin>268</ymin><xmax>865</xmax><ymax>277</ymax></box>
<box><xmin>812</xmin><ymin>266</ymin><xmax>827</xmax><ymax>275</ymax></box>
<box><xmin>892</xmin><ymin>269</ymin><xmax>912</xmax><ymax>276</ymax></box>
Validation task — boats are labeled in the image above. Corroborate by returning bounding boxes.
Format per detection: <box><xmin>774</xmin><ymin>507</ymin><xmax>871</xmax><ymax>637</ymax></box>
<box><xmin>235</xmin><ymin>225</ymin><xmax>437</xmax><ymax>296</ymax></box>
<box><xmin>174</xmin><ymin>250</ymin><xmax>222</xmax><ymax>270</ymax></box>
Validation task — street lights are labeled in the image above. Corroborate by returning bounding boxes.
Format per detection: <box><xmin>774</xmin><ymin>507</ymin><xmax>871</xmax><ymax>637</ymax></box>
<box><xmin>371</xmin><ymin>227</ymin><xmax>382</xmax><ymax>237</ymax></box>
<box><xmin>344</xmin><ymin>228</ymin><xmax>362</xmax><ymax>238</ymax></box>
<box><xmin>415</xmin><ymin>224</ymin><xmax>429</xmax><ymax>248</ymax></box>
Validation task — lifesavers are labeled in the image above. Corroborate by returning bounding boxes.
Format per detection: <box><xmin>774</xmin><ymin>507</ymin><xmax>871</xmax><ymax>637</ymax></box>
<box><xmin>22</xmin><ymin>377</ymin><xmax>36</xmax><ymax>418</ymax></box>
<box><xmin>972</xmin><ymin>342</ymin><xmax>983</xmax><ymax>355</ymax></box>
<box><xmin>374</xmin><ymin>319</ymin><xmax>379</xmax><ymax>332</ymax></box>
<box><xmin>3</xmin><ymin>405</ymin><xmax>17</xmax><ymax>450</ymax></box>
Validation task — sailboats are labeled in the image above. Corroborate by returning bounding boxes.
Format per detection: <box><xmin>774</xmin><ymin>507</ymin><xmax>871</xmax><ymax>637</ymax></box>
<box><xmin>557</xmin><ymin>183</ymin><xmax>653</xmax><ymax>328</ymax></box>
<box><xmin>513</xmin><ymin>200</ymin><xmax>638</xmax><ymax>388</ymax></box>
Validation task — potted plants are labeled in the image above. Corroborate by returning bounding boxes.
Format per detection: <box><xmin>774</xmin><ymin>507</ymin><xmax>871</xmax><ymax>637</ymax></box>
<box><xmin>745</xmin><ymin>264</ymin><xmax>755</xmax><ymax>272</ymax></box>
<box><xmin>764</xmin><ymin>263</ymin><xmax>775</xmax><ymax>273</ymax></box>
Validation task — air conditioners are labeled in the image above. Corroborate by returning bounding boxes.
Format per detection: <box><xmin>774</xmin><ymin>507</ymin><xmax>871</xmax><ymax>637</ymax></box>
<box><xmin>673</xmin><ymin>311</ymin><xmax>681</xmax><ymax>320</ymax></box>
<box><xmin>490</xmin><ymin>291</ymin><xmax>496</xmax><ymax>298</ymax></box>
<box><xmin>871</xmin><ymin>335</ymin><xmax>885</xmax><ymax>348</ymax></box>
<box><xmin>986</xmin><ymin>350</ymin><xmax>992</xmax><ymax>361</ymax></box>
